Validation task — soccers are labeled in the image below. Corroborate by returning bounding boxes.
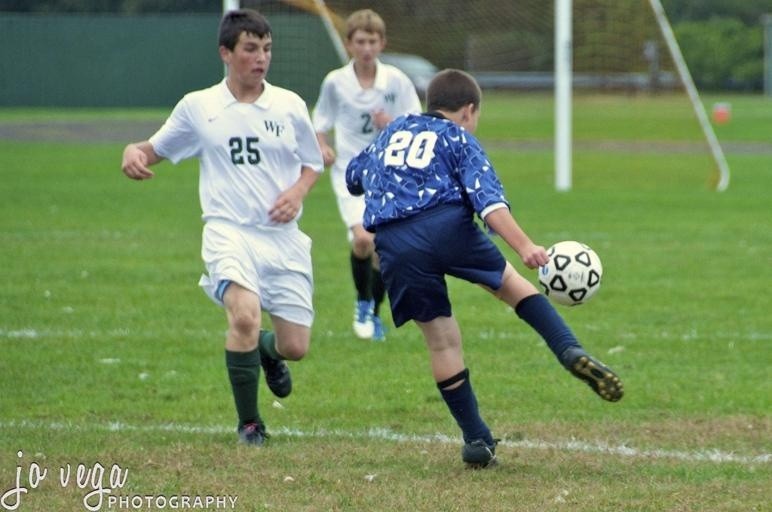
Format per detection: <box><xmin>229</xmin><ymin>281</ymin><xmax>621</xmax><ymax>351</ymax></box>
<box><xmin>538</xmin><ymin>241</ymin><xmax>602</xmax><ymax>306</ymax></box>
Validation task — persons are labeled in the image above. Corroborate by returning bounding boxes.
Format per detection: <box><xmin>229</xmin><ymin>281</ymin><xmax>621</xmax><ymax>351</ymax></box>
<box><xmin>122</xmin><ymin>9</ymin><xmax>325</xmax><ymax>449</ymax></box>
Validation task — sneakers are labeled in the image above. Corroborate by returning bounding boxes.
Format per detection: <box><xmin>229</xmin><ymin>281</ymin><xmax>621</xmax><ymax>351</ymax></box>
<box><xmin>259</xmin><ymin>329</ymin><xmax>292</xmax><ymax>398</ymax></box>
<box><xmin>353</xmin><ymin>297</ymin><xmax>387</xmax><ymax>343</ymax></box>
<box><xmin>565</xmin><ymin>347</ymin><xmax>624</xmax><ymax>402</ymax></box>
<box><xmin>238</xmin><ymin>420</ymin><xmax>265</xmax><ymax>445</ymax></box>
<box><xmin>461</xmin><ymin>439</ymin><xmax>497</xmax><ymax>468</ymax></box>
